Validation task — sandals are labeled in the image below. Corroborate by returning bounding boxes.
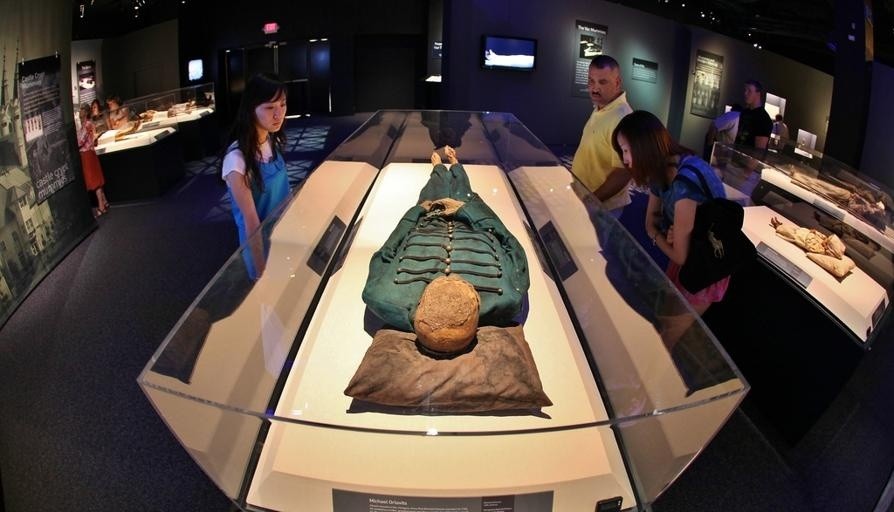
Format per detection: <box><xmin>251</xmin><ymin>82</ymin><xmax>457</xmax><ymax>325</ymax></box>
<box><xmin>94</xmin><ymin>202</ymin><xmax>110</xmax><ymax>218</ymax></box>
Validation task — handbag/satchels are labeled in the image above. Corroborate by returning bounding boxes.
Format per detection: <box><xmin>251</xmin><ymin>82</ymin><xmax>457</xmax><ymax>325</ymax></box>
<box><xmin>679</xmin><ymin>199</ymin><xmax>758</xmax><ymax>295</ymax></box>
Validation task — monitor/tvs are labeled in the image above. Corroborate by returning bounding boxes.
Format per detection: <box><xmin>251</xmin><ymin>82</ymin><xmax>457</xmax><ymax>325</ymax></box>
<box><xmin>479</xmin><ymin>34</ymin><xmax>538</xmax><ymax>73</ymax></box>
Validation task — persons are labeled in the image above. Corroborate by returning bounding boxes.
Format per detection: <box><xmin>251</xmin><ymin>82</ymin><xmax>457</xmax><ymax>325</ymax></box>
<box><xmin>703</xmin><ymin>79</ymin><xmax>790</xmax><ymax>175</ymax></box>
<box><xmin>573</xmin><ymin>55</ymin><xmax>633</xmax><ymax>251</ymax></box>
<box><xmin>222</xmin><ymin>75</ymin><xmax>288</xmax><ymax>286</ymax></box>
<box><xmin>771</xmin><ymin>165</ymin><xmax>888</xmax><ymax>260</ymax></box>
<box><xmin>611</xmin><ymin>110</ymin><xmax>731</xmax><ymax>351</ymax></box>
<box><xmin>77</xmin><ymin>95</ymin><xmax>129</xmax><ymax>218</ymax></box>
<box><xmin>363</xmin><ymin>145</ymin><xmax>527</xmax><ymax>353</ymax></box>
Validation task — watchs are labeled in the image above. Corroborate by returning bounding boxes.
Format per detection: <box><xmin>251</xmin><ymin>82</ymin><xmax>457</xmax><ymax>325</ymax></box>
<box><xmin>652</xmin><ymin>230</ymin><xmax>662</xmax><ymax>246</ymax></box>
